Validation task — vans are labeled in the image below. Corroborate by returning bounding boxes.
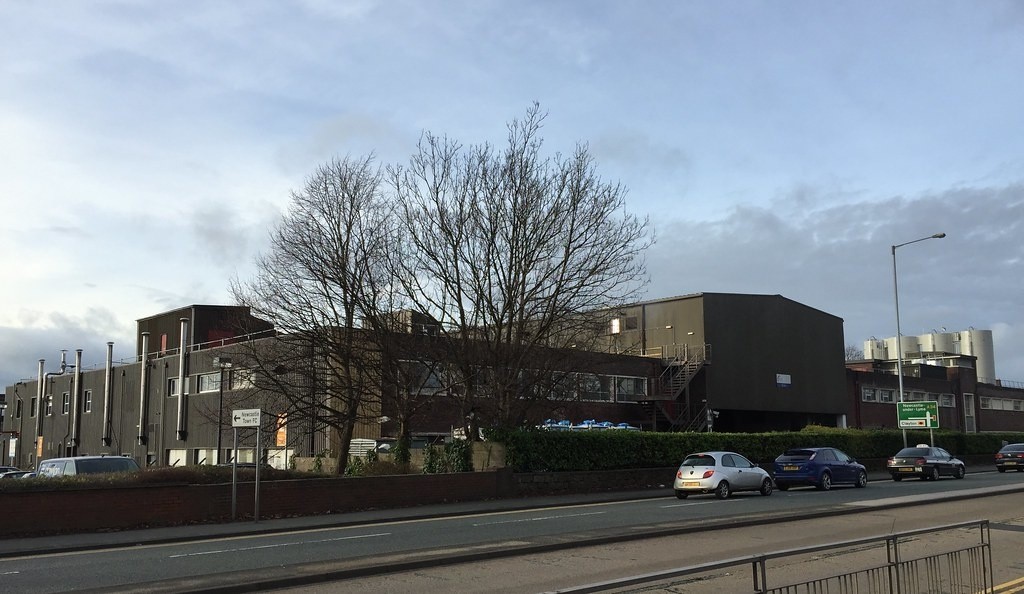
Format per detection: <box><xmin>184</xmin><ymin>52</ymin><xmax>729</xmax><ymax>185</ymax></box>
<box><xmin>36</xmin><ymin>454</ymin><xmax>143</xmax><ymax>477</ymax></box>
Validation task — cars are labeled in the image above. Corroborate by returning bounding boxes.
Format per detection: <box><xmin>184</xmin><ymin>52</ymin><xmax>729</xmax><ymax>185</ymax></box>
<box><xmin>0</xmin><ymin>467</ymin><xmax>36</xmax><ymax>478</ymax></box>
<box><xmin>773</xmin><ymin>447</ymin><xmax>867</xmax><ymax>490</ymax></box>
<box><xmin>673</xmin><ymin>452</ymin><xmax>773</xmax><ymax>499</ymax></box>
<box><xmin>994</xmin><ymin>444</ymin><xmax>1024</xmax><ymax>472</ymax></box>
<box><xmin>887</xmin><ymin>444</ymin><xmax>966</xmax><ymax>480</ymax></box>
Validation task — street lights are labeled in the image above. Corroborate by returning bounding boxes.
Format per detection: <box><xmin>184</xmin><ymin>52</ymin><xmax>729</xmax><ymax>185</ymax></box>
<box><xmin>892</xmin><ymin>233</ymin><xmax>934</xmax><ymax>448</ymax></box>
